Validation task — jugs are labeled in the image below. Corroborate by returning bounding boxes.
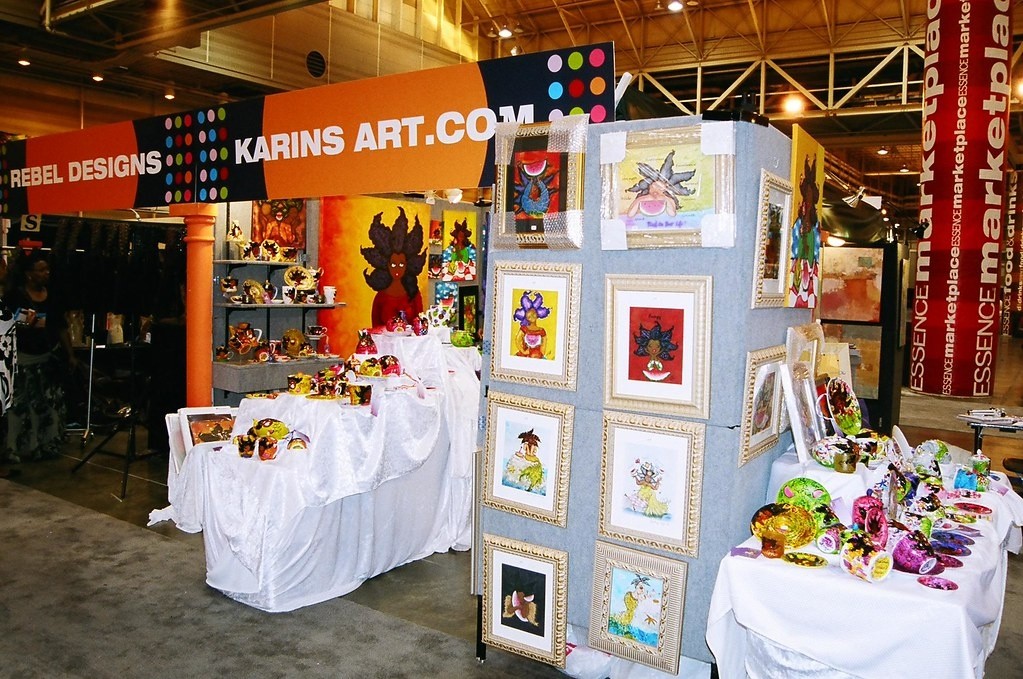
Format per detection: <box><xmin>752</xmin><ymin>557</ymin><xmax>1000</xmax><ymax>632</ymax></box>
<box><xmin>356</xmin><ymin>328</ymin><xmax>378</xmax><ymax>354</ymax></box>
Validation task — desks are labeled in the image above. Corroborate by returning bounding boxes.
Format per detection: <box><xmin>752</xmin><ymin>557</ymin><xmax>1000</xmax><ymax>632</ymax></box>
<box><xmin>966</xmin><ymin>421</ymin><xmax>1023</xmax><ymax>454</ymax></box>
<box><xmin>704</xmin><ymin>443</ymin><xmax>1023</xmax><ymax>679</ymax></box>
<box><xmin>72</xmin><ymin>344</ymin><xmax>150</xmax><ymax>436</ymax></box>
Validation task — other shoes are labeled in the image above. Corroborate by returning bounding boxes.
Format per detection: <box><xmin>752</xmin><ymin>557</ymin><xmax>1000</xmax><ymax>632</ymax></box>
<box><xmin>36</xmin><ymin>446</ymin><xmax>59</xmax><ymax>460</ymax></box>
<box><xmin>8</xmin><ymin>450</ymin><xmax>27</xmax><ymax>465</ymax></box>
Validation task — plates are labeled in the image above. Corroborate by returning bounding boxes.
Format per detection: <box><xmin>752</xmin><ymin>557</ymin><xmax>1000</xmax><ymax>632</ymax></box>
<box><xmin>281</xmin><ymin>329</ymin><xmax>306</xmax><ymax>356</ymax></box>
<box><xmin>810</xmin><ymin>377</ymin><xmax>914</xmax><ymax>481</ymax></box>
<box><xmin>284</xmin><ymin>266</ymin><xmax>314</xmax><ymax>290</ymax></box>
<box><xmin>261</xmin><ymin>239</ymin><xmax>281</xmax><ymax>262</ymax></box>
<box><xmin>271</xmin><ymin>300</ymin><xmax>284</xmax><ymax>304</ymax></box>
<box><xmin>306</xmin><ymin>335</ymin><xmax>325</xmax><ymax>340</ymax></box>
<box><xmin>750</xmin><ymin>477</ymin><xmax>991</xmax><ymax>592</ymax></box>
<box><xmin>242</xmin><ymin>279</ymin><xmax>271</xmax><ymax>304</ymax></box>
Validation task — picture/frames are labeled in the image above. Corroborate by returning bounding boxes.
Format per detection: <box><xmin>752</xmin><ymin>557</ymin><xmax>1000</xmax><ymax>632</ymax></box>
<box><xmin>178</xmin><ymin>405</ymin><xmax>239</xmax><ymax>455</ymax></box>
<box><xmin>599</xmin><ymin>120</ymin><xmax>738</xmax><ymax>250</ymax></box>
<box><xmin>598</xmin><ymin>410</ymin><xmax>706</xmax><ymax>559</ymax></box>
<box><xmin>481</xmin><ymin>532</ymin><xmax>570</xmax><ymax>669</ymax></box>
<box><xmin>588</xmin><ymin>540</ymin><xmax>688</xmax><ymax>677</ymax></box>
<box><xmin>737</xmin><ymin>343</ymin><xmax>787</xmax><ymax>468</ymax></box>
<box><xmin>750</xmin><ymin>168</ymin><xmax>793</xmax><ymax>310</ymax></box>
<box><xmin>480</xmin><ymin>392</ymin><xmax>575</xmax><ymax>528</ymax></box>
<box><xmin>490</xmin><ymin>260</ymin><xmax>584</xmax><ymax>391</ymax></box>
<box><xmin>780</xmin><ymin>361</ymin><xmax>829</xmax><ymax>466</ymax></box>
<box><xmin>602</xmin><ymin>274</ymin><xmax>713</xmax><ymax>420</ymax></box>
<box><xmin>495</xmin><ymin>113</ymin><xmax>591</xmax><ymax>249</ymax></box>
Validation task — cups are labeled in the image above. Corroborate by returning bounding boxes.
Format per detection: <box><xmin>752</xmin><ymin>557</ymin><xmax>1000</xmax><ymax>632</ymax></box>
<box><xmin>413</xmin><ymin>317</ymin><xmax>428</xmax><ymax>336</ymax></box>
<box><xmin>237</xmin><ymin>434</ymin><xmax>307</xmax><ymax>461</ymax></box>
<box><xmin>386</xmin><ymin>311</ymin><xmax>407</xmax><ymax>333</ymax></box>
<box><xmin>256</xmin><ymin>343</ymin><xmax>275</xmax><ymax>362</ymax></box>
<box><xmin>281</xmin><ymin>286</ymin><xmax>297</xmax><ymax>305</ymax></box>
<box><xmin>323</xmin><ymin>286</ymin><xmax>337</xmax><ymax>305</ymax></box>
<box><xmin>287</xmin><ymin>355</ymin><xmax>401</xmax><ymax>406</ymax></box>
<box><xmin>307</xmin><ymin>325</ymin><xmax>327</xmax><ymax>335</ymax></box>
<box><xmin>762</xmin><ymin>441</ymin><xmax>991</xmax><ymax>583</ymax></box>
<box><xmin>230</xmin><ymin>296</ymin><xmax>243</xmax><ymax>304</ymax></box>
<box><xmin>228</xmin><ymin>332</ymin><xmax>252</xmax><ymax>355</ymax></box>
<box><xmin>238</xmin><ymin>327</ymin><xmax>262</xmax><ymax>347</ymax></box>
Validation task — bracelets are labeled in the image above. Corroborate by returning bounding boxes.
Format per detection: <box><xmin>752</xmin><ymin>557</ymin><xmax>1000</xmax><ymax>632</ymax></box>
<box><xmin>31</xmin><ymin>316</ymin><xmax>41</xmax><ymax>327</ymax></box>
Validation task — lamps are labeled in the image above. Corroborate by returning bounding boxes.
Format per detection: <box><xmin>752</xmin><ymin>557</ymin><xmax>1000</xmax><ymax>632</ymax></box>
<box><xmin>653</xmin><ymin>0</ymin><xmax>665</xmax><ymax>11</ymax></box>
<box><xmin>165</xmin><ymin>86</ymin><xmax>177</xmax><ymax>101</ymax></box>
<box><xmin>218</xmin><ymin>93</ymin><xmax>229</xmax><ymax>105</ymax></box>
<box><xmin>825</xmin><ymin>169</ymin><xmax>865</xmax><ymax>209</ymax></box>
<box><xmin>92</xmin><ymin>72</ymin><xmax>106</xmax><ymax>81</ymax></box>
<box><xmin>667</xmin><ymin>0</ymin><xmax>683</xmax><ymax>12</ymax></box>
<box><xmin>444</xmin><ymin>189</ymin><xmax>463</xmax><ymax>205</ymax></box>
<box><xmin>513</xmin><ymin>22</ymin><xmax>524</xmax><ymax>34</ymax></box>
<box><xmin>16</xmin><ymin>58</ymin><xmax>33</xmax><ymax>68</ymax></box>
<box><xmin>425</xmin><ymin>190</ymin><xmax>438</xmax><ymax>206</ymax></box>
<box><xmin>498</xmin><ymin>25</ymin><xmax>513</xmax><ymax>37</ymax></box>
<box><xmin>487</xmin><ymin>27</ymin><xmax>497</xmax><ymax>38</ymax></box>
<box><xmin>884</xmin><ymin>200</ymin><xmax>930</xmax><ymax>240</ymax></box>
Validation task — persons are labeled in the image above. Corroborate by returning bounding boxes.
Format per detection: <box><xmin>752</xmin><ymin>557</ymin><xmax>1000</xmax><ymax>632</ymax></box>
<box><xmin>140</xmin><ymin>284</ymin><xmax>187</xmax><ymax>345</ymax></box>
<box><xmin>10</xmin><ymin>259</ymin><xmax>77</xmax><ymax>462</ymax></box>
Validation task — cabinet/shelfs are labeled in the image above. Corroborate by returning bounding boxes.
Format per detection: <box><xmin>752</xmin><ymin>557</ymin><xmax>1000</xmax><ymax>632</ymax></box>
<box><xmin>170</xmin><ymin>331</ymin><xmax>480</xmax><ymax>614</ymax></box>
<box><xmin>213</xmin><ymin>258</ymin><xmax>347</xmax><ymax>394</ymax></box>
